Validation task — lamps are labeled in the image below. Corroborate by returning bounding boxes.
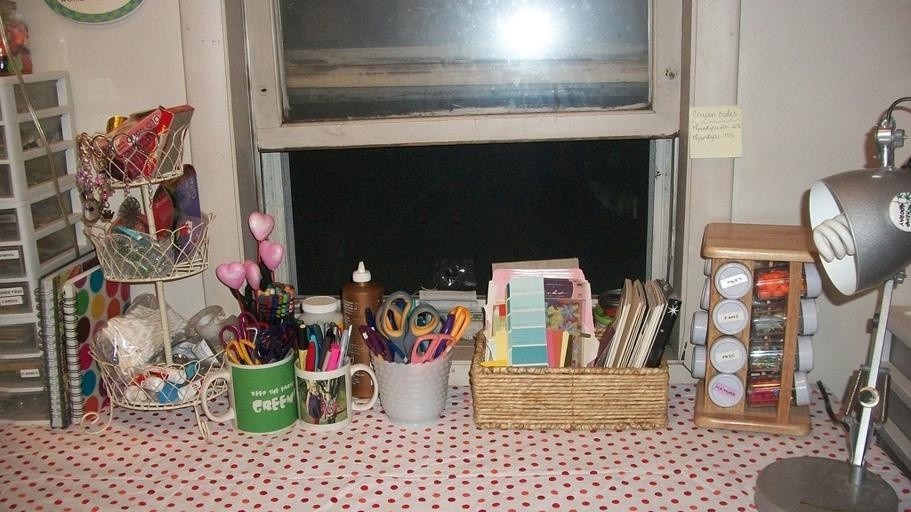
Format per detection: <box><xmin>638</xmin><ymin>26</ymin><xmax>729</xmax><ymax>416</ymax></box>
<box><xmin>754</xmin><ymin>96</ymin><xmax>911</xmax><ymax>511</ymax></box>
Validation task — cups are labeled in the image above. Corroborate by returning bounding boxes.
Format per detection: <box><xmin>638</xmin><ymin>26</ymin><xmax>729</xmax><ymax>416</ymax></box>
<box><xmin>200</xmin><ymin>348</ymin><xmax>298</xmax><ymax>434</ymax></box>
<box><xmin>435</xmin><ymin>258</ymin><xmax>476</xmax><ymax>291</ymax></box>
<box><xmin>294</xmin><ymin>357</ymin><xmax>378</xmax><ymax>431</ymax></box>
<box><xmin>374</xmin><ymin>354</ymin><xmax>456</xmax><ymax>428</ymax></box>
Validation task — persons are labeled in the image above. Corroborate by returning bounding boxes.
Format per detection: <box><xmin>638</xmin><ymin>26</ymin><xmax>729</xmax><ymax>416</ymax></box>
<box><xmin>321</xmin><ymin>392</ymin><xmax>337</xmax><ymax>424</ymax></box>
<box><xmin>306</xmin><ymin>383</ymin><xmax>323</xmax><ymax>424</ymax></box>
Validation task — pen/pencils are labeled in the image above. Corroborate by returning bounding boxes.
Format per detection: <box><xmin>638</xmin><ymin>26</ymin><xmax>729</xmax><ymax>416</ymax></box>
<box><xmin>186</xmin><ymin>358</ymin><xmax>220</xmax><ymax>368</ymax></box>
<box><xmin>296</xmin><ymin>319</ymin><xmax>353</xmax><ymax>372</ymax></box>
<box><xmin>245</xmin><ymin>282</ymin><xmax>295</xmax><ymax>318</ymax></box>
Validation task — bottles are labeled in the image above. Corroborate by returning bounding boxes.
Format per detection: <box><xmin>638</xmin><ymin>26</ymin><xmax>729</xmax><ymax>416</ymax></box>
<box><xmin>751</xmin><ymin>298</ymin><xmax>818</xmax><ymax>338</ymax></box>
<box><xmin>746</xmin><ymin>371</ymin><xmax>810</xmax><ymax>408</ymax></box>
<box><xmin>341</xmin><ymin>262</ymin><xmax>383</xmax><ymax>398</ymax></box>
<box><xmin>298</xmin><ymin>294</ymin><xmax>344</xmax><ymax>332</ymax></box>
<box><xmin>749</xmin><ymin>335</ymin><xmax>814</xmax><ymax>373</ymax></box>
<box><xmin>754</xmin><ymin>263</ymin><xmax>822</xmax><ymax>300</ymax></box>
<box><xmin>0</xmin><ymin>1</ymin><xmax>32</xmax><ymax>75</ymax></box>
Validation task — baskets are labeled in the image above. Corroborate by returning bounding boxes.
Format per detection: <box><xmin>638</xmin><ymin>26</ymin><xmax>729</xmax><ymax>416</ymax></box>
<box><xmin>83</xmin><ymin>212</ymin><xmax>217</xmax><ymax>283</ymax></box>
<box><xmin>78</xmin><ymin>124</ymin><xmax>190</xmax><ymax>188</ymax></box>
<box><xmin>469</xmin><ymin>327</ymin><xmax>669</xmax><ymax>430</ymax></box>
<box><xmin>88</xmin><ymin>341</ymin><xmax>228</xmax><ymax>411</ymax></box>
<box><xmin>245</xmin><ymin>297</ymin><xmax>294</xmax><ymax>326</ymax></box>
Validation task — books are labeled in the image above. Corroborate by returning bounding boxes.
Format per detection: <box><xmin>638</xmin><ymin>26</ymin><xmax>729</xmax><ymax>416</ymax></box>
<box><xmin>477</xmin><ymin>258</ymin><xmax>671</xmax><ymax>368</ymax></box>
<box><xmin>59</xmin><ymin>264</ymin><xmax>131</xmax><ymax>426</ymax></box>
<box><xmin>34</xmin><ymin>248</ymin><xmax>105</xmax><ymax>429</ymax></box>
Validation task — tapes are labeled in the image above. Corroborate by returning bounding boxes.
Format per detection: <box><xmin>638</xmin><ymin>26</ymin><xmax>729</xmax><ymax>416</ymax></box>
<box><xmin>185</xmin><ymin>305</ymin><xmax>226</xmax><ymax>339</ymax></box>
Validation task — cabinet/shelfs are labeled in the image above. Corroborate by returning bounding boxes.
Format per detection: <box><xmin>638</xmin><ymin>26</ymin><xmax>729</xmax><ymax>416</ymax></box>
<box><xmin>692</xmin><ymin>223</ymin><xmax>819</xmax><ymax>437</ymax></box>
<box><xmin>80</xmin><ymin>125</ymin><xmax>228</xmax><ymax>442</ymax></box>
<box><xmin>0</xmin><ymin>71</ymin><xmax>90</xmax><ymax>427</ymax></box>
<box><xmin>876</xmin><ymin>307</ymin><xmax>911</xmax><ymax>472</ymax></box>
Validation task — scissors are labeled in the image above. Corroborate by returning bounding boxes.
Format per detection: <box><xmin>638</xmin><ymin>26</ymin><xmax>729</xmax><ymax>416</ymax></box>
<box><xmin>358</xmin><ymin>291</ymin><xmax>471</xmax><ymax>364</ymax></box>
<box><xmin>218</xmin><ymin>312</ymin><xmax>297</xmax><ymax>365</ymax></box>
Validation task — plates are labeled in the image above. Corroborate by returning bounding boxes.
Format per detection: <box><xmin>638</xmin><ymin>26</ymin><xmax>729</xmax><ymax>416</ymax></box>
<box><xmin>43</xmin><ymin>1</ymin><xmax>146</xmax><ymax>25</ymax></box>
